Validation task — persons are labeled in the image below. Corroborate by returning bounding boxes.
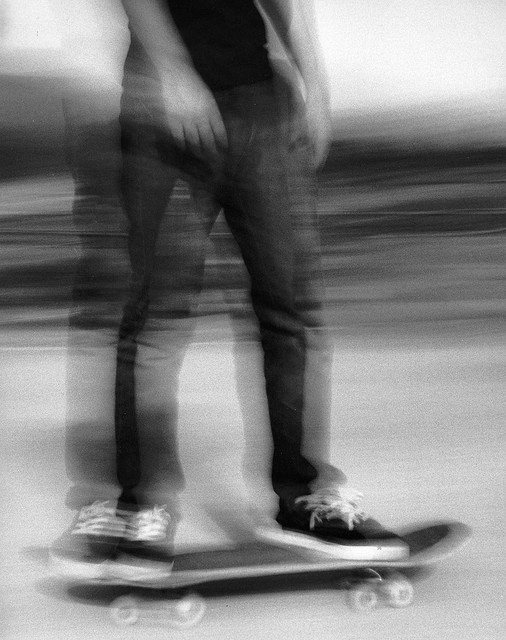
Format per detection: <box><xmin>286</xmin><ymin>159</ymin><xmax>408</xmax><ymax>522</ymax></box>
<box><xmin>112</xmin><ymin>0</ymin><xmax>411</xmax><ymax>582</ymax></box>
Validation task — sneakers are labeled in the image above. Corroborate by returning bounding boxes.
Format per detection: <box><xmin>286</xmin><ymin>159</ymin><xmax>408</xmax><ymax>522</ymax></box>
<box><xmin>61</xmin><ymin>506</ymin><xmax>179</xmax><ymax>580</ymax></box>
<box><xmin>253</xmin><ymin>486</ymin><xmax>413</xmax><ymax>563</ymax></box>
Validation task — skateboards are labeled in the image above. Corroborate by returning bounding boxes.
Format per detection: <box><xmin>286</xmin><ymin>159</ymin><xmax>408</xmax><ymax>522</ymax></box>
<box><xmin>20</xmin><ymin>521</ymin><xmax>477</xmax><ymax>619</ymax></box>
